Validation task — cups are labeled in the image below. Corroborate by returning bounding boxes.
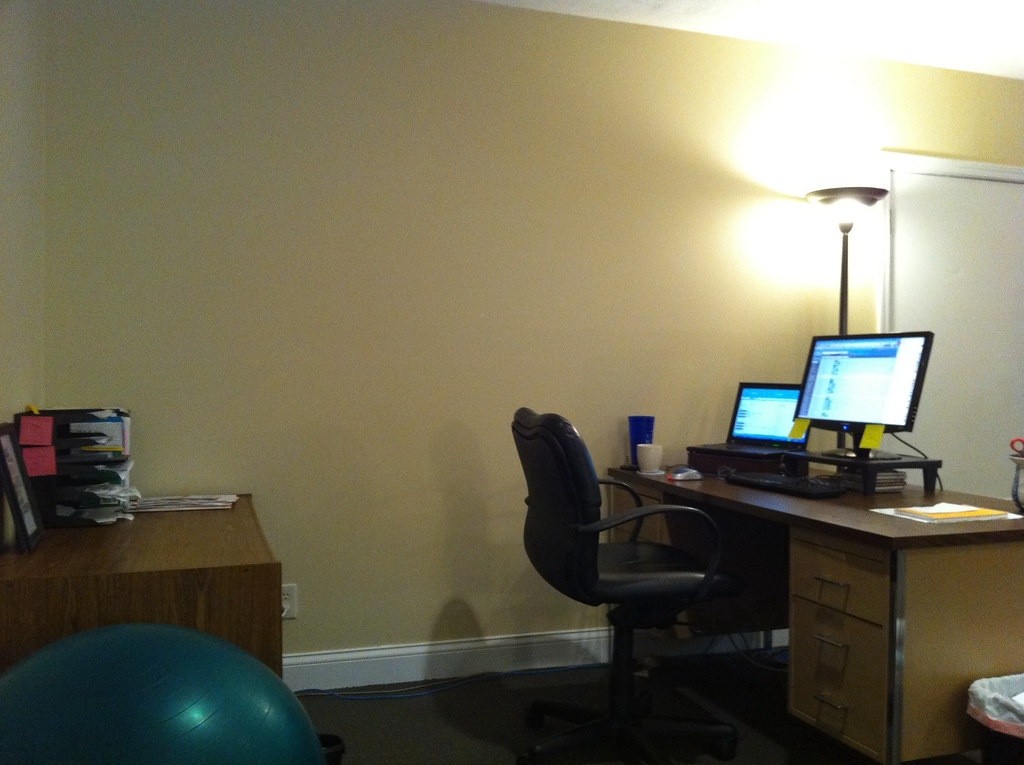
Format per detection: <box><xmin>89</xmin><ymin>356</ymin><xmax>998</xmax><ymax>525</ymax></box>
<box><xmin>628</xmin><ymin>415</ymin><xmax>656</xmax><ymax>465</ymax></box>
<box><xmin>637</xmin><ymin>444</ymin><xmax>662</xmax><ymax>473</ymax></box>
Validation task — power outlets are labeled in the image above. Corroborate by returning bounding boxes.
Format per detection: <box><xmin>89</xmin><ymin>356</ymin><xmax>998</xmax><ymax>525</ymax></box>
<box><xmin>281</xmin><ymin>584</ymin><xmax>297</xmax><ymax>618</ymax></box>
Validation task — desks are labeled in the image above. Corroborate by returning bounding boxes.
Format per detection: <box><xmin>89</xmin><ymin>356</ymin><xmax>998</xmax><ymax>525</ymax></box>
<box><xmin>0</xmin><ymin>494</ymin><xmax>282</xmax><ymax>684</ymax></box>
<box><xmin>605</xmin><ymin>469</ymin><xmax>1024</xmax><ymax>765</ymax></box>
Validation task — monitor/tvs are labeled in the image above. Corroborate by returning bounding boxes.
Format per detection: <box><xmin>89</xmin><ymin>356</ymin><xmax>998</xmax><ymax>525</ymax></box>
<box><xmin>793</xmin><ymin>330</ymin><xmax>934</xmax><ymax>461</ymax></box>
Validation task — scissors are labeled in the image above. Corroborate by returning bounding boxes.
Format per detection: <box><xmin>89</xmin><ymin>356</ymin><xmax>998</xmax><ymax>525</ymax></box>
<box><xmin>1009</xmin><ymin>437</ymin><xmax>1024</xmax><ymax>457</ymax></box>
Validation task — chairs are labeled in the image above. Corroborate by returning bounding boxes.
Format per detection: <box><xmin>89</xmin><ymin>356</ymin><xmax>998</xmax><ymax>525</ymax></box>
<box><xmin>511</xmin><ymin>406</ymin><xmax>743</xmax><ymax>765</ymax></box>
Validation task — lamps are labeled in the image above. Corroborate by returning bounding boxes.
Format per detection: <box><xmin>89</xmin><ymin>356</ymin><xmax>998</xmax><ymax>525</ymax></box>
<box><xmin>803</xmin><ymin>185</ymin><xmax>891</xmax><ymax>481</ymax></box>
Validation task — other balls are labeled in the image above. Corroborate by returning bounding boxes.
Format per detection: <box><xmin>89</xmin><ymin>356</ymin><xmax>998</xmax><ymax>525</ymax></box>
<box><xmin>0</xmin><ymin>622</ymin><xmax>326</xmax><ymax>764</ymax></box>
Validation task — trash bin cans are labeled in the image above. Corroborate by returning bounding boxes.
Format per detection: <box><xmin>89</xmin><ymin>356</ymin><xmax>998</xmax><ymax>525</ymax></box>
<box><xmin>965</xmin><ymin>673</ymin><xmax>1024</xmax><ymax>765</ymax></box>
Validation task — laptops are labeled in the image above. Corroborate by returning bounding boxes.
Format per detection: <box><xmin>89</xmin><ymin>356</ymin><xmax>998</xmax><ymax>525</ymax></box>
<box><xmin>686</xmin><ymin>381</ymin><xmax>811</xmax><ymax>460</ymax></box>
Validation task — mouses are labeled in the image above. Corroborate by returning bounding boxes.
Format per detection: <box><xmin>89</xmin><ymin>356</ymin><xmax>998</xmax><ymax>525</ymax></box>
<box><xmin>666</xmin><ymin>465</ymin><xmax>703</xmax><ymax>480</ymax></box>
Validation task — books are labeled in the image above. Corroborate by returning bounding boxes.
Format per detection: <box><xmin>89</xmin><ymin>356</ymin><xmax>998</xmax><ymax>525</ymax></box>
<box><xmin>895</xmin><ymin>504</ymin><xmax>1008</xmax><ymax>521</ymax></box>
<box><xmin>839</xmin><ymin>468</ymin><xmax>906</xmax><ymax>493</ymax></box>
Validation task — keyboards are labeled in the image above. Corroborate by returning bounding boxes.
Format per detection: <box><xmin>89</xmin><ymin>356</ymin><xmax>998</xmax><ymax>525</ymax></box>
<box><xmin>725</xmin><ymin>471</ymin><xmax>847</xmax><ymax>499</ymax></box>
